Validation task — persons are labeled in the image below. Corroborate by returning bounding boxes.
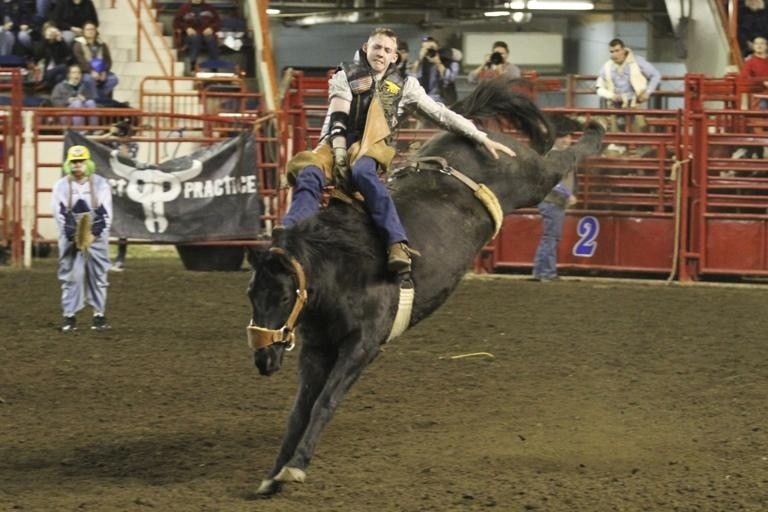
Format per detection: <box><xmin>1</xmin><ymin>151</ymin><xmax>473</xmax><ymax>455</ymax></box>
<box><xmin>467</xmin><ymin>41</ymin><xmax>521</xmax><ymax>86</ymax></box>
<box><xmin>269</xmin><ymin>26</ymin><xmax>518</xmax><ymax>276</ymax></box>
<box><xmin>738</xmin><ymin>36</ymin><xmax>767</xmax><ymax>110</ymax></box>
<box><xmin>597</xmin><ymin>38</ymin><xmax>661</xmax><ymax>133</ymax></box>
<box><xmin>50</xmin><ymin>145</ymin><xmax>116</xmax><ymax>331</ymax></box>
<box><xmin>531</xmin><ymin>125</ymin><xmax>576</xmax><ymax>281</ymax></box>
<box><xmin>99</xmin><ymin>120</ymin><xmax>140</xmax><ymax>268</ymax></box>
<box><xmin>392</xmin><ymin>38</ymin><xmax>462</xmax><ymax>152</ymax></box>
<box><xmin>1</xmin><ymin>1</ymin><xmax>138</xmax><ymax>141</ymax></box>
<box><xmin>174</xmin><ymin>0</ymin><xmax>221</xmax><ymax>72</ymax></box>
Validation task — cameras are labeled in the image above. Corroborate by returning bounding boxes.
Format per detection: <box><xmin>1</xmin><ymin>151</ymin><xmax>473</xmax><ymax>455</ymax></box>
<box><xmin>424</xmin><ymin>49</ymin><xmax>436</xmax><ymax>61</ymax></box>
<box><xmin>484</xmin><ymin>52</ymin><xmax>504</xmax><ymax>69</ymax></box>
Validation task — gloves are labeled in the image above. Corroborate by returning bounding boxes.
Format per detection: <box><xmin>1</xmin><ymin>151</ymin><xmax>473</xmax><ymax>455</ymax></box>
<box><xmin>332</xmin><ymin>149</ymin><xmax>352</xmax><ymax>186</ymax></box>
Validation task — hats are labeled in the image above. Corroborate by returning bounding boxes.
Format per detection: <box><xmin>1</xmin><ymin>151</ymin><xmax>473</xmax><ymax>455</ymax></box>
<box><xmin>67</xmin><ymin>145</ymin><xmax>89</xmax><ymax>160</ymax></box>
<box><xmin>89</xmin><ymin>59</ymin><xmax>110</xmax><ymax>72</ymax></box>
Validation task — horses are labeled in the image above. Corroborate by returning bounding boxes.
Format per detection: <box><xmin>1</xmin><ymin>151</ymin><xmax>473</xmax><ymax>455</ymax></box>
<box><xmin>244</xmin><ymin>74</ymin><xmax>612</xmax><ymax>495</ymax></box>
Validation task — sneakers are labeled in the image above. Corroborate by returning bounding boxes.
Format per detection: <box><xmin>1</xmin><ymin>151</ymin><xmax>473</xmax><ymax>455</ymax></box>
<box><xmin>62</xmin><ymin>315</ymin><xmax>77</xmax><ymax>331</ymax></box>
<box><xmin>389</xmin><ymin>243</ymin><xmax>412</xmax><ymax>269</ymax></box>
<box><xmin>92</xmin><ymin>314</ymin><xmax>111</xmax><ymax>330</ymax></box>
